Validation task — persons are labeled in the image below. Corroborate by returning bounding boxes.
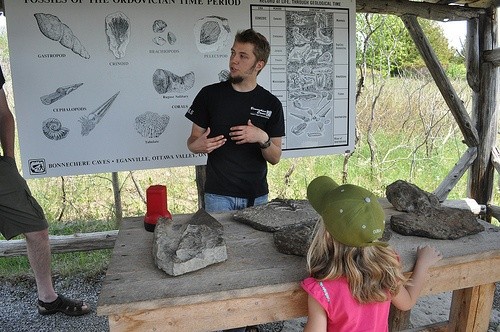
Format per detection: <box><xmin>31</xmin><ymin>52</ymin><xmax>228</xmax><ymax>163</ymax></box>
<box><xmin>302</xmin><ymin>175</ymin><xmax>443</xmax><ymax>331</ymax></box>
<box><xmin>0</xmin><ymin>63</ymin><xmax>93</xmax><ymax>316</ymax></box>
<box><xmin>184</xmin><ymin>27</ymin><xmax>286</xmax><ymax>331</ymax></box>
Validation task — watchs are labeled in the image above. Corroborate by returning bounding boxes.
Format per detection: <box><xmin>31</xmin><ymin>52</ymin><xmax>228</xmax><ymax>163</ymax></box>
<box><xmin>260</xmin><ymin>136</ymin><xmax>271</xmax><ymax>148</ymax></box>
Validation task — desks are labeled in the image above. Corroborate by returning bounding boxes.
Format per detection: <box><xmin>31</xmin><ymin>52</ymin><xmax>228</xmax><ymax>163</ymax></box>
<box><xmin>94</xmin><ymin>204</ymin><xmax>500</xmax><ymax>332</ymax></box>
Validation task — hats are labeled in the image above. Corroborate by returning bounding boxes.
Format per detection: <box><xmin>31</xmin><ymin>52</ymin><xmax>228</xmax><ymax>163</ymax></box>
<box><xmin>307</xmin><ymin>176</ymin><xmax>389</xmax><ymax>247</ymax></box>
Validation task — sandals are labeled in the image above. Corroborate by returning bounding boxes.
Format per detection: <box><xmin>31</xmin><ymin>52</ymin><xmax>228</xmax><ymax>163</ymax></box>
<box><xmin>38</xmin><ymin>293</ymin><xmax>91</xmax><ymax>316</ymax></box>
